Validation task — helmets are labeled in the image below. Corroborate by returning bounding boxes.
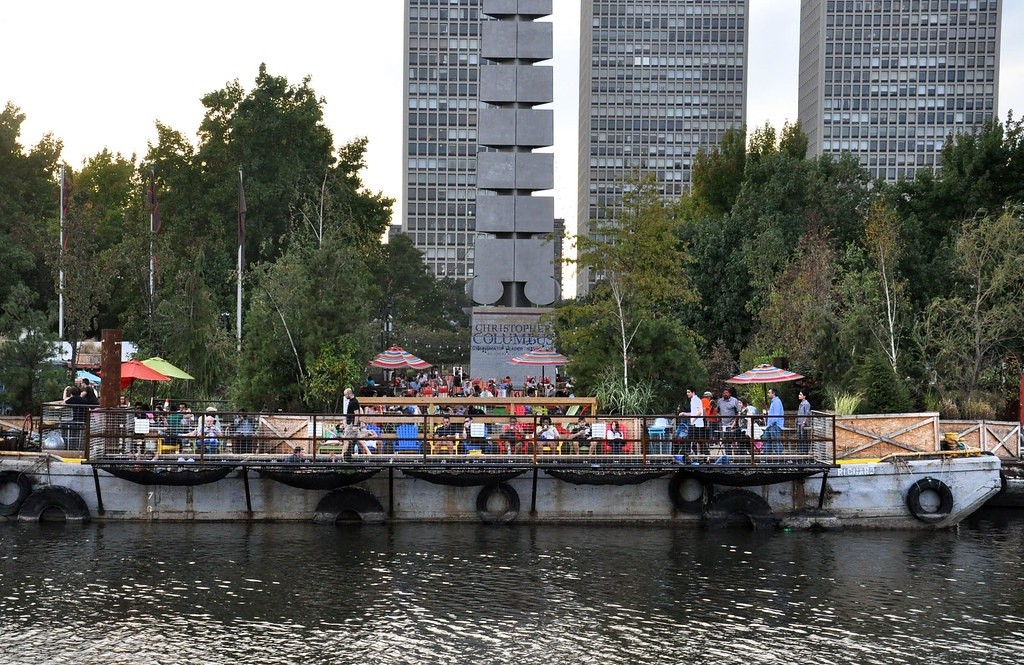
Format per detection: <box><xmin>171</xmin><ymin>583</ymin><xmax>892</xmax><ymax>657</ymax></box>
<box><xmin>703</xmin><ymin>392</ymin><xmax>713</xmax><ymax>397</ymax></box>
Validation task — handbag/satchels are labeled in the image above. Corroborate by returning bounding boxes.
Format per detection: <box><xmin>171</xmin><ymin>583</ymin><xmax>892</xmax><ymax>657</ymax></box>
<box><xmin>88</xmin><ymin>395</ymin><xmax>100</xmax><ymax>410</ymax></box>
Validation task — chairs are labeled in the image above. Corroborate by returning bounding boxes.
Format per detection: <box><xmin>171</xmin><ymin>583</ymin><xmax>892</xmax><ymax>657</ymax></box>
<box><xmin>393</xmin><ymin>424</ymin><xmax>422</xmax><ymax>454</ymax></box>
<box><xmin>532</xmin><ymin>425</ymin><xmax>563</xmax><ymax>456</ymax></box>
<box><xmin>319</xmin><ymin>423</ymin><xmax>345</xmax><ymax>453</ymax></box>
<box><xmin>567</xmin><ymin>423</ymin><xmax>597</xmax><ymax>456</ymax></box>
<box><xmin>357</xmin><ymin>426</ymin><xmax>385</xmax><ymax>453</ymax></box>
<box><xmin>601</xmin><ymin>424</ymin><xmax>633</xmax><ymax>455</ymax></box>
<box><xmin>374</xmin><ymin>375</ymin><xmax>592</xmax><ymax>416</ymax></box>
<box><xmin>135</xmin><ymin>413</ymin><xmax>259</xmax><ymax>455</ymax></box>
<box><xmin>462</xmin><ymin>427</ymin><xmax>493</xmax><ymax>453</ymax></box>
<box><xmin>648</xmin><ymin>418</ymin><xmax>667</xmax><ymax>439</ymax></box>
<box><xmin>497</xmin><ymin>425</ymin><xmax>529</xmax><ymax>455</ymax></box>
<box><xmin>429</xmin><ymin>424</ymin><xmax>459</xmax><ymax>455</ymax></box>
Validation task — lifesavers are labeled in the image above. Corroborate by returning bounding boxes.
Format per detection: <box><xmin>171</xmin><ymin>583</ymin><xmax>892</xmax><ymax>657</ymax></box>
<box><xmin>698</xmin><ymin>487</ymin><xmax>777</xmax><ymax>529</ymax></box>
<box><xmin>0</xmin><ymin>470</ymin><xmax>33</xmax><ymax>517</ymax></box>
<box><xmin>311</xmin><ymin>485</ymin><xmax>386</xmax><ymax>521</ymax></box>
<box><xmin>16</xmin><ymin>485</ymin><xmax>92</xmax><ymax>521</ymax></box>
<box><xmin>668</xmin><ymin>469</ymin><xmax>715</xmax><ymax>515</ymax></box>
<box><xmin>906</xmin><ymin>477</ymin><xmax>953</xmax><ymax>524</ymax></box>
<box><xmin>475</xmin><ymin>481</ymin><xmax>521</xmax><ymax>526</ymax></box>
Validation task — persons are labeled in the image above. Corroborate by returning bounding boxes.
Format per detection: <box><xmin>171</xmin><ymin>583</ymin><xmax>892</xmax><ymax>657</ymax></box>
<box><xmin>462</xmin><ymin>417</ymin><xmax>492</xmax><ymax>454</ymax></box>
<box><xmin>361</xmin><ymin>367</ymin><xmax>574</xmax><ymax>414</ymax></box>
<box><xmin>291</xmin><ymin>388</ymin><xmax>377</xmax><ymax>465</ymax></box>
<box><xmin>607</xmin><ymin>421</ymin><xmax>625</xmax><ymax>454</ymax></box>
<box><xmin>570</xmin><ymin>418</ymin><xmax>598</xmax><ymax>455</ymax></box>
<box><xmin>433</xmin><ymin>417</ymin><xmax>455</xmax><ymax>454</ymax></box>
<box><xmin>678</xmin><ymin>387</ymin><xmax>812</xmax><ymax>458</ymax></box>
<box><xmin>63</xmin><ymin>378</ymin><xmax>256</xmax><ymax>454</ymax></box>
<box><xmin>500</xmin><ymin>417</ymin><xmax>525</xmax><ymax>455</ymax></box>
<box><xmin>532</xmin><ymin>417</ymin><xmax>560</xmax><ymax>454</ymax></box>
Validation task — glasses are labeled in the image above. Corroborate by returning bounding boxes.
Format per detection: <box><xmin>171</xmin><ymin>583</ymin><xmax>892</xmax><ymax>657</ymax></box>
<box><xmin>136</xmin><ymin>407</ymin><xmax>142</xmax><ymax>409</ymax></box>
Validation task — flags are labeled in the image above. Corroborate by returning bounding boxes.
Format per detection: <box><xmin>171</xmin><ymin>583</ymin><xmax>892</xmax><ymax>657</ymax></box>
<box><xmin>237</xmin><ymin>171</ymin><xmax>247</xmax><ymax>273</ymax></box>
<box><xmin>61</xmin><ymin>177</ymin><xmax>69</xmax><ymax>252</ymax></box>
<box><xmin>145</xmin><ymin>169</ymin><xmax>162</xmax><ymax>234</ymax></box>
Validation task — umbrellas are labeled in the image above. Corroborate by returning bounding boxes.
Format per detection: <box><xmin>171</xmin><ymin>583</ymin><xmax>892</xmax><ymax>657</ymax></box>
<box><xmin>75</xmin><ymin>356</ymin><xmax>195</xmax><ymax>406</ymax></box>
<box><xmin>367</xmin><ymin>343</ymin><xmax>434</xmax><ymax>387</ymax></box>
<box><xmin>505</xmin><ymin>344</ymin><xmax>575</xmax><ymax>387</ymax></box>
<box><xmin>724</xmin><ymin>363</ymin><xmax>805</xmax><ymax>409</ymax></box>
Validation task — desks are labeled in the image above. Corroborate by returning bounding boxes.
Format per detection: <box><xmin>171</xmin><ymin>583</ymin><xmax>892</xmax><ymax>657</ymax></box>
<box><xmin>149</xmin><ymin>427</ymin><xmax>168</xmax><ymax>431</ymax></box>
<box><xmin>713</xmin><ymin>430</ymin><xmax>736</xmax><ymax>456</ymax></box>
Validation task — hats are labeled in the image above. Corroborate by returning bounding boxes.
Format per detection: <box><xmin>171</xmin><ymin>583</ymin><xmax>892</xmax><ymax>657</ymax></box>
<box><xmin>206</xmin><ymin>406</ymin><xmax>218</xmax><ymax>412</ymax></box>
